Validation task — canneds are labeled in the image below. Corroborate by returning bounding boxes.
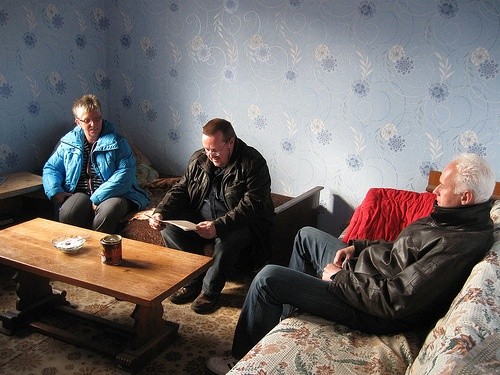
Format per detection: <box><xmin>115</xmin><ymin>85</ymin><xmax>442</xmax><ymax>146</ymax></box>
<box><xmin>100</xmin><ymin>234</ymin><xmax>122</xmax><ymax>265</ymax></box>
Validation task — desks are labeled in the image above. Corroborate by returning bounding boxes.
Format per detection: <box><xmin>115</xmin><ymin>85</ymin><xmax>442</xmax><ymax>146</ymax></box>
<box><xmin>0</xmin><ymin>171</ymin><xmax>43</xmax><ymax>217</ymax></box>
<box><xmin>0</xmin><ymin>217</ymin><xmax>213</xmax><ymax>373</ymax></box>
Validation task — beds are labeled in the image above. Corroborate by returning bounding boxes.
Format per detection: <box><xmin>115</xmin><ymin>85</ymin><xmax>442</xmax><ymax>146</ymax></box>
<box><xmin>29</xmin><ymin>160</ymin><xmax>324</xmax><ymax>279</ymax></box>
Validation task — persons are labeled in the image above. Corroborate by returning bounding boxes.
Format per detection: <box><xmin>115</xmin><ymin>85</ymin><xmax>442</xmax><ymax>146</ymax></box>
<box><xmin>205</xmin><ymin>153</ymin><xmax>497</xmax><ymax>375</ymax></box>
<box><xmin>42</xmin><ymin>94</ymin><xmax>153</xmax><ymax>234</ymax></box>
<box><xmin>149</xmin><ymin>118</ymin><xmax>274</xmax><ymax>312</ymax></box>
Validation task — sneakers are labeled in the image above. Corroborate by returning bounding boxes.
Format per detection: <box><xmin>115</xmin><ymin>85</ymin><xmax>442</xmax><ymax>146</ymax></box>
<box><xmin>207</xmin><ymin>357</ymin><xmax>240</xmax><ymax>375</ymax></box>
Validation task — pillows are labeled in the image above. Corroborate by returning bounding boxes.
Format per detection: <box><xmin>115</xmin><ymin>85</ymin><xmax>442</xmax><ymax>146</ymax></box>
<box><xmin>134</xmin><ymin>150</ymin><xmax>159</xmax><ymax>188</ymax></box>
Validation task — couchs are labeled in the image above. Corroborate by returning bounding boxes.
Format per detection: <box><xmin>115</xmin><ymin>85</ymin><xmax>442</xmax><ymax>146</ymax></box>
<box><xmin>224</xmin><ymin>200</ymin><xmax>500</xmax><ymax>375</ymax></box>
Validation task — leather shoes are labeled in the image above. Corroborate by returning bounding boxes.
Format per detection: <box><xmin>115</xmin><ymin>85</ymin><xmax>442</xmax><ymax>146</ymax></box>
<box><xmin>190</xmin><ymin>293</ymin><xmax>221</xmax><ymax>312</ymax></box>
<box><xmin>168</xmin><ymin>283</ymin><xmax>199</xmax><ymax>304</ymax></box>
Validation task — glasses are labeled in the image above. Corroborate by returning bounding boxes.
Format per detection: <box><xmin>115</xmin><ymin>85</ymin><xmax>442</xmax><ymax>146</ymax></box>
<box><xmin>201</xmin><ymin>143</ymin><xmax>231</xmax><ymax>156</ymax></box>
<box><xmin>76</xmin><ymin>114</ymin><xmax>101</xmax><ymax>124</ymax></box>
